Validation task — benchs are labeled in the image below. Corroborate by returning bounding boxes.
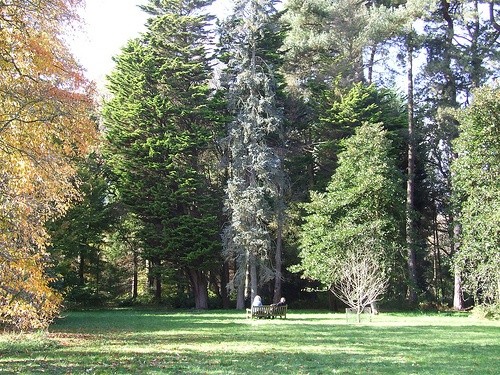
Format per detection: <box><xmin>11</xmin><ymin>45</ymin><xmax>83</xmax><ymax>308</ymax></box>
<box><xmin>252</xmin><ymin>305</ymin><xmax>288</xmax><ymax>319</ymax></box>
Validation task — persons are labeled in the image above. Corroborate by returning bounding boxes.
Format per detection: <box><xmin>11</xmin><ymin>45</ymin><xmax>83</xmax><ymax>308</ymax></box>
<box><xmin>252</xmin><ymin>294</ymin><xmax>267</xmax><ymax>317</ymax></box>
<box><xmin>267</xmin><ymin>296</ymin><xmax>287</xmax><ymax>316</ymax></box>
